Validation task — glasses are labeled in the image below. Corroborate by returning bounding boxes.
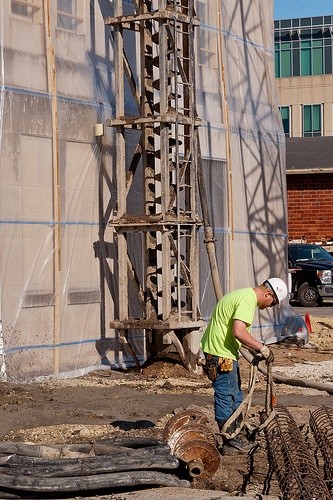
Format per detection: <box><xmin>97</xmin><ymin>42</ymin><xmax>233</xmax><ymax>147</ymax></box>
<box><xmin>271</xmin><ymin>295</ymin><xmax>277</xmax><ymax>306</ymax></box>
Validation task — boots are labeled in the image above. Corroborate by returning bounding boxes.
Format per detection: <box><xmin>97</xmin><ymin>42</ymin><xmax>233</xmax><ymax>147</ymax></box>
<box><xmin>218</xmin><ymin>421</ymin><xmax>251</xmax><ymax>456</ymax></box>
<box><xmin>235</xmin><ymin>412</ymin><xmax>250</xmax><ymax>434</ymax></box>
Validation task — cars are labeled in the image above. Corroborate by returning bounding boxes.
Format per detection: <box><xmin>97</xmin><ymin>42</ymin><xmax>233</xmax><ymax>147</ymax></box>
<box><xmin>288</xmin><ymin>243</ymin><xmax>333</xmax><ymax>307</ymax></box>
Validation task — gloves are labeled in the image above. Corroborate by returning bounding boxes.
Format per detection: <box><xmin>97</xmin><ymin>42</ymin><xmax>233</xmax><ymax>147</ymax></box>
<box><xmin>250</xmin><ymin>343</ymin><xmax>274</xmax><ymax>366</ymax></box>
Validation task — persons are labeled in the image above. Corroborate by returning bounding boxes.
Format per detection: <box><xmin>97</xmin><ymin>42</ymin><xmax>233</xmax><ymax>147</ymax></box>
<box><xmin>200</xmin><ymin>277</ymin><xmax>287</xmax><ymax>455</ymax></box>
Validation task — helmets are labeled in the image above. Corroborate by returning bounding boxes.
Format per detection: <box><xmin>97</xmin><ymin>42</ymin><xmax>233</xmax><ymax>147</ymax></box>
<box><xmin>266</xmin><ymin>278</ymin><xmax>288</xmax><ymax>308</ymax></box>
